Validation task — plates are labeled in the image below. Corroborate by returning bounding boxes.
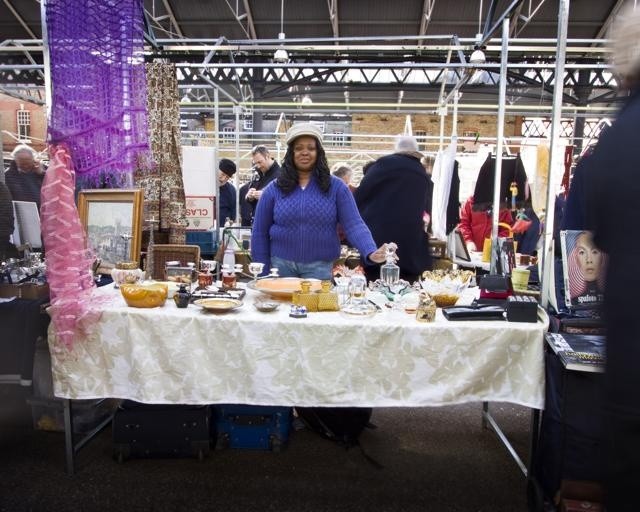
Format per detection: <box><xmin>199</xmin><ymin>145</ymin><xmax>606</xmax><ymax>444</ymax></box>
<box><xmin>247</xmin><ymin>277</ymin><xmax>326</xmax><ymax>298</ymax></box>
<box><xmin>193</xmin><ymin>297</ymin><xmax>244</xmax><ymax>313</ymax></box>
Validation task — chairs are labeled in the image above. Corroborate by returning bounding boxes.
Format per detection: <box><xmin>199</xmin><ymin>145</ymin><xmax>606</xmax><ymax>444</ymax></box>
<box><xmin>146</xmin><ymin>244</ymin><xmax>200</xmax><ymax>283</ymax></box>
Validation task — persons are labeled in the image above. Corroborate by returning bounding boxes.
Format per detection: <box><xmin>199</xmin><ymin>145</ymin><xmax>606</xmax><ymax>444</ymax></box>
<box><xmin>458</xmin><ymin>194</ymin><xmax>515</xmax><ymax>254</ymax></box>
<box><xmin>239</xmin><ymin>166</ymin><xmax>261</xmax><ymax>226</ymax></box>
<box><xmin>565</xmin><ymin>7</ymin><xmax>640</xmax><ymax>512</ymax></box>
<box><xmin>570</xmin><ymin>232</ymin><xmax>606</xmax><ymax>304</ymax></box>
<box><xmin>244</xmin><ymin>144</ymin><xmax>281</xmax><ymax>207</ymax></box>
<box><xmin>353</xmin><ymin>135</ymin><xmax>435</xmax><ymax>286</ymax></box>
<box><xmin>5</xmin><ymin>144</ymin><xmax>47</xmax><ymax>217</ymax></box>
<box><xmin>214</xmin><ymin>159</ymin><xmax>237</xmax><ymax>227</ymax></box>
<box><xmin>329</xmin><ymin>162</ymin><xmax>357</xmax><ymax>250</ymax></box>
<box><xmin>251</xmin><ymin>122</ymin><xmax>399</xmax><ymax>285</ymax></box>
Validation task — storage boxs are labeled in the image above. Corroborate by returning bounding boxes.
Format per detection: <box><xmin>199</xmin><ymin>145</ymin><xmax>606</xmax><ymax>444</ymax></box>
<box><xmin>0</xmin><ymin>280</ymin><xmax>48</xmax><ymax>301</ymax></box>
<box><xmin>25</xmin><ymin>394</ymin><xmax>117</xmax><ymax>436</ymax></box>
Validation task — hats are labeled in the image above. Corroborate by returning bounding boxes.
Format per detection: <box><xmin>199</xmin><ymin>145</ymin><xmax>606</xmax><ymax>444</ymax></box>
<box><xmin>219</xmin><ymin>158</ymin><xmax>237</xmax><ymax>177</ymax></box>
<box><xmin>284</xmin><ymin>123</ymin><xmax>325</xmax><ymax>143</ymax></box>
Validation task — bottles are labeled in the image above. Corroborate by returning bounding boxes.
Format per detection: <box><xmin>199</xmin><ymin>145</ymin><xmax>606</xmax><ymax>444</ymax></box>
<box><xmin>222</xmin><ymin>250</ymin><xmax>234</xmax><ymax>267</ymax></box>
<box><xmin>315</xmin><ymin>280</ymin><xmax>338</xmax><ymax>311</ymax></box>
<box><xmin>380</xmin><ymin>246</ymin><xmax>399</xmax><ymax>286</ymax></box>
<box><xmin>292</xmin><ymin>282</ymin><xmax>317</xmax><ymax>312</ymax></box>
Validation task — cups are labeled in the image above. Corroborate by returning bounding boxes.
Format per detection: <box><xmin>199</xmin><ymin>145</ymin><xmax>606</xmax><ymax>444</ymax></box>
<box><xmin>201</xmin><ymin>259</ymin><xmax>215</xmax><ymax>271</ymax></box>
<box><xmin>249</xmin><ymin>262</ymin><xmax>264</xmax><ymax>282</ymax></box>
<box><xmin>346</xmin><ymin>276</ymin><xmax>366</xmax><ymax>304</ymax></box>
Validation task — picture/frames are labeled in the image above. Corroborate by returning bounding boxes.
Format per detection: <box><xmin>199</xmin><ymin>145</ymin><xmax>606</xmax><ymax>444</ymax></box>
<box><xmin>77</xmin><ymin>188</ymin><xmax>145</xmax><ymax>274</ymax></box>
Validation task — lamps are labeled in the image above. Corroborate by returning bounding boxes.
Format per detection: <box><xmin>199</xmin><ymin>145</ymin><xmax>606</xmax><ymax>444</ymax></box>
<box><xmin>469</xmin><ymin>32</ymin><xmax>486</xmax><ymax>64</ymax></box>
<box><xmin>273</xmin><ymin>32</ymin><xmax>290</xmax><ymax>63</ymax></box>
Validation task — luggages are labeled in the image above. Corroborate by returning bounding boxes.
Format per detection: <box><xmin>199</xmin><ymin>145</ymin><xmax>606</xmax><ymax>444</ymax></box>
<box><xmin>211</xmin><ymin>404</ymin><xmax>292</xmax><ymax>454</ymax></box>
<box><xmin>109</xmin><ymin>399</ymin><xmax>210</xmax><ymax>461</ymax></box>
